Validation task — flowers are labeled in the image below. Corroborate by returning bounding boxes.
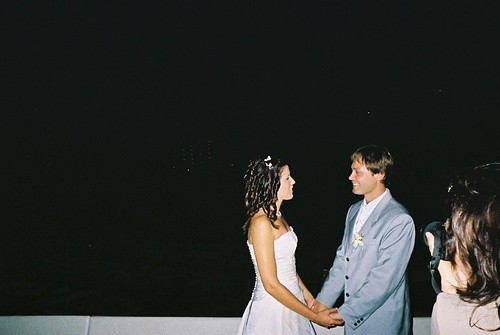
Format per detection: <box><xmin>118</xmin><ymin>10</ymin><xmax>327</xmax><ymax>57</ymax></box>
<box><xmin>352</xmin><ymin>233</ymin><xmax>363</xmax><ymax>252</ymax></box>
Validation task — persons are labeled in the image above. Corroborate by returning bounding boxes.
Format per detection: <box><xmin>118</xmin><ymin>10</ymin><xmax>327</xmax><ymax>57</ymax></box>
<box><xmin>310</xmin><ymin>142</ymin><xmax>416</xmax><ymax>335</ymax></box>
<box><xmin>238</xmin><ymin>155</ymin><xmax>343</xmax><ymax>335</ymax></box>
<box><xmin>424</xmin><ymin>162</ymin><xmax>500</xmax><ymax>335</ymax></box>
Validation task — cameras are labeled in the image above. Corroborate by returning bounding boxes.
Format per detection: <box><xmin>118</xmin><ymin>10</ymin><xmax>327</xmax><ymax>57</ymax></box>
<box><xmin>420</xmin><ymin>220</ymin><xmax>454</xmax><ymax>263</ymax></box>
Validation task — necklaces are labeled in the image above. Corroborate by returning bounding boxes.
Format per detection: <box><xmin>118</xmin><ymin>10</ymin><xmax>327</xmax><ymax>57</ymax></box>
<box><xmin>275</xmin><ymin>210</ymin><xmax>282</xmax><ymax>217</ymax></box>
